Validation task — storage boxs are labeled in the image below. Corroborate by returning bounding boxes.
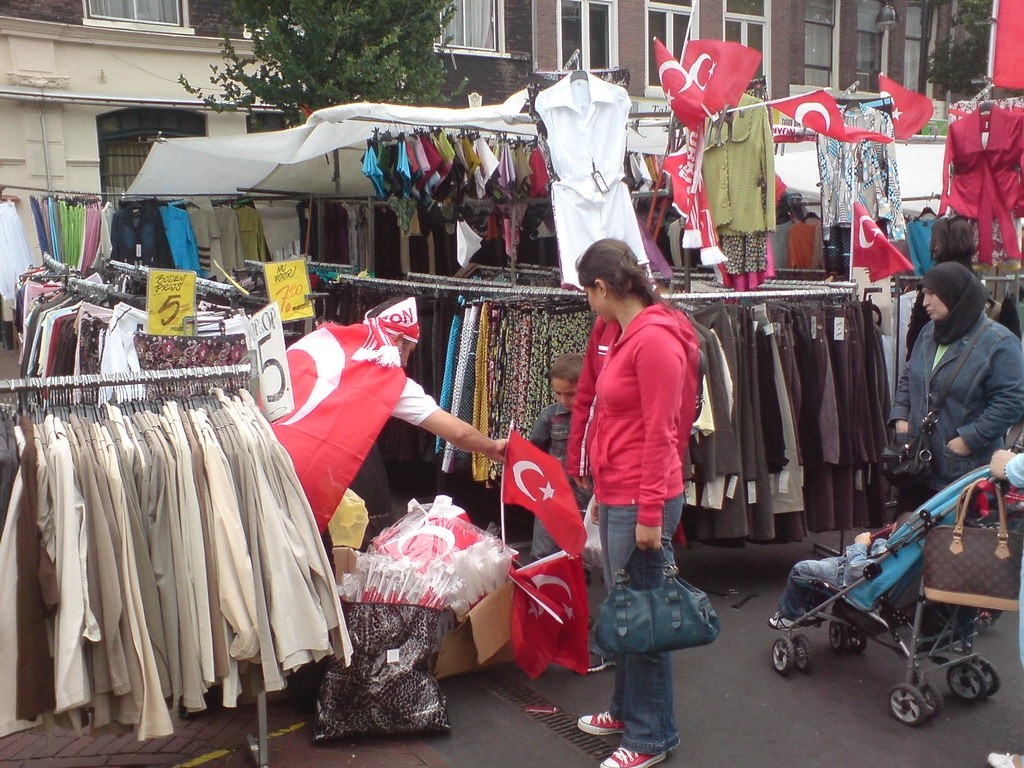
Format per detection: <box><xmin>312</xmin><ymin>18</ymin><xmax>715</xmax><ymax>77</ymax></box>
<box><xmin>425</xmin><ymin>580</ymin><xmax>517</xmax><ymax>678</ymax></box>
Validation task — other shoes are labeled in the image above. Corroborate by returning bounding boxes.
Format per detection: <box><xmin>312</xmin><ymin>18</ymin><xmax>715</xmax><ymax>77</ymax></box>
<box><xmin>574</xmin><ymin>650</ymin><xmax>616</xmax><ymax>674</ymax></box>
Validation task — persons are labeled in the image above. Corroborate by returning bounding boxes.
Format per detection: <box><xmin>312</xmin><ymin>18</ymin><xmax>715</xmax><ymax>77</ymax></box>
<box><xmin>987</xmin><ymin>450</ymin><xmax>1024</xmax><ymax>768</ymax></box>
<box><xmin>264</xmin><ymin>297</ymin><xmax>507</xmax><ymax>714</ymax></box>
<box><xmin>892</xmin><ymin>216</ymin><xmax>976</xmax><ymax>521</ymax></box>
<box><xmin>578</xmin><ymin>240</ymin><xmax>698</xmax><ymax>768</ymax></box>
<box><xmin>768</xmin><ymin>511</ymin><xmax>914</xmax><ymax>629</ymax></box>
<box><xmin>886</xmin><ymin>261</ymin><xmax>1024</xmax><ymax>662</ymax></box>
<box><xmin>565</xmin><ymin>315</ymin><xmax>618</xmax><ymax>672</ymax></box>
<box><xmin>526</xmin><ymin>352</ymin><xmax>593</xmax><ymax>562</ymax></box>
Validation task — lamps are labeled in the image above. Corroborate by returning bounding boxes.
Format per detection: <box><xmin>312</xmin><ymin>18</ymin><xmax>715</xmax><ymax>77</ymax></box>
<box><xmin>874</xmin><ymin>0</ymin><xmax>900</xmax><ymax>31</ymax></box>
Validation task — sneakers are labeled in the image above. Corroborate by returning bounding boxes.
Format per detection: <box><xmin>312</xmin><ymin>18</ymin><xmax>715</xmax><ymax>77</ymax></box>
<box><xmin>600</xmin><ymin>745</ymin><xmax>668</xmax><ymax>768</ymax></box>
<box><xmin>578</xmin><ymin>711</ymin><xmax>623</xmax><ymax>735</ymax></box>
<box><xmin>766</xmin><ymin>613</ymin><xmax>802</xmax><ymax>629</ymax></box>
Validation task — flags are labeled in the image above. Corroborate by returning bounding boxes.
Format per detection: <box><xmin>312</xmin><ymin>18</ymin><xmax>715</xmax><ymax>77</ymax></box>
<box><xmin>853</xmin><ymin>203</ymin><xmax>914</xmax><ymax>282</ymax></box>
<box><xmin>877</xmin><ymin>73</ymin><xmax>934</xmax><ymax>140</ymax></box>
<box><xmin>502</xmin><ymin>431</ymin><xmax>586</xmax><ymax>558</ymax></box>
<box><xmin>770</xmin><ymin>89</ymin><xmax>891</xmax><ymax>145</ymax></box>
<box><xmin>652</xmin><ymin>35</ymin><xmax>762</xmax><ymax>133</ymax></box>
<box><xmin>509</xmin><ymin>549</ymin><xmax>590</xmax><ymax>680</ymax></box>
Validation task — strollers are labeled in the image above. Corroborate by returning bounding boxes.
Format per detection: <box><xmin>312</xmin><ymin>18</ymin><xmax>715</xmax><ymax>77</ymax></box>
<box><xmin>773</xmin><ymin>451</ymin><xmax>1024</xmax><ymax>727</ymax></box>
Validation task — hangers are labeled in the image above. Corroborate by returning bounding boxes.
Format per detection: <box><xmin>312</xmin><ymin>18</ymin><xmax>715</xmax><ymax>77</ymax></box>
<box><xmin>979</xmin><ymin>89</ymin><xmax>994</xmax><ymax>113</ymax></box>
<box><xmin>570</xmin><ymin>54</ymin><xmax>588</xmax><ymax>82</ymax></box>
<box><xmin>366</xmin><ymin>119</ymin><xmax>539</xmax><ymax>150</ymax></box>
<box><xmin>0</xmin><ymin>182</ymin><xmax>859</xmax><ymax>426</ymax></box>
<box><xmin>844</xmin><ymin>91</ymin><xmax>861</xmax><ymax>113</ymax></box>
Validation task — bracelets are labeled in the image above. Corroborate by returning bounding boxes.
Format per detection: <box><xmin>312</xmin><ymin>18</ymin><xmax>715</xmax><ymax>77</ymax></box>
<box><xmin>1004</xmin><ymin>464</ymin><xmax>1008</xmax><ymax>481</ymax></box>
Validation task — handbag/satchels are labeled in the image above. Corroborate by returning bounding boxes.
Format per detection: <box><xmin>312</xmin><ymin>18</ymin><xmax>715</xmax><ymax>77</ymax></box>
<box><xmin>880</xmin><ymin>410</ymin><xmax>937</xmax><ymax>478</ymax></box>
<box><xmin>922</xmin><ymin>478</ymin><xmax>1024</xmax><ymax>610</ymax></box>
<box><xmin>314</xmin><ymin>601</ymin><xmax>451</xmax><ymax>738</ymax></box>
<box><xmin>593</xmin><ymin>541</ymin><xmax>720</xmax><ymax>653</ymax></box>
